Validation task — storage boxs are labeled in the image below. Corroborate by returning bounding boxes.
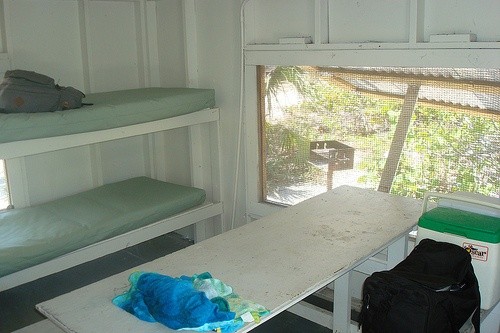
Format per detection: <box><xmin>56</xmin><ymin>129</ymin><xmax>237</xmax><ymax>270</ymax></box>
<box><xmin>417</xmin><ymin>206</ymin><xmax>500</xmax><ymax>310</ymax></box>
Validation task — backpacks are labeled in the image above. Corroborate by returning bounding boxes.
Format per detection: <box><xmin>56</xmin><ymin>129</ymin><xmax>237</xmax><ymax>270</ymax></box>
<box><xmin>0</xmin><ymin>70</ymin><xmax>93</xmax><ymax>113</ymax></box>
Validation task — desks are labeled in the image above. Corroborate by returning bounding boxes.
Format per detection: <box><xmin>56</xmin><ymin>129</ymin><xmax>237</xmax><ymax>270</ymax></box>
<box><xmin>34</xmin><ymin>185</ymin><xmax>439</xmax><ymax>333</ymax></box>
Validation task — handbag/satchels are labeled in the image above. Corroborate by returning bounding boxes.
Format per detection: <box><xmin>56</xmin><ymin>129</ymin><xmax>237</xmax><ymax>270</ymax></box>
<box><xmin>357</xmin><ymin>239</ymin><xmax>480</xmax><ymax>333</ymax></box>
